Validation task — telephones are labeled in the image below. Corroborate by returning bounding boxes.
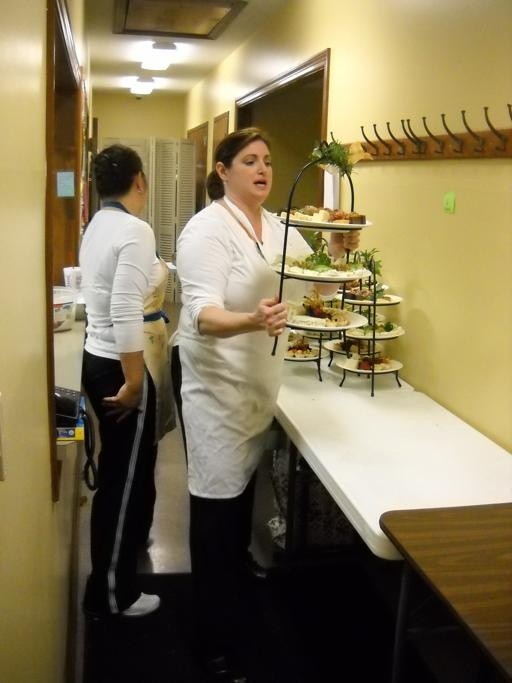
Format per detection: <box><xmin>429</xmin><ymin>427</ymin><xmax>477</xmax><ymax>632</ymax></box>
<box><xmin>54</xmin><ymin>385</ymin><xmax>81</xmax><ymax>427</ymax></box>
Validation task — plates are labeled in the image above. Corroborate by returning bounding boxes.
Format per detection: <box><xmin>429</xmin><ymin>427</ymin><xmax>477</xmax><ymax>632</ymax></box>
<box><xmin>271</xmin><ymin>210</ymin><xmax>405</xmax><ymax>375</ymax></box>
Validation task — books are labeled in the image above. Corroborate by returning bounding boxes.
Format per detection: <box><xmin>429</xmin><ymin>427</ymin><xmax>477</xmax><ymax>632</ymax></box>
<box><xmin>55</xmin><ymin>394</ymin><xmax>86</xmax><ymax>440</ymax></box>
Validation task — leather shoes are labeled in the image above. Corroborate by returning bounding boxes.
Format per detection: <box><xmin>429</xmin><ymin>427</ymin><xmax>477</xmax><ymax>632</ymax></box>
<box><xmin>214</xmin><ymin>657</ymin><xmax>247</xmax><ymax>682</ymax></box>
<box><xmin>248</xmin><ymin>558</ymin><xmax>271</xmax><ymax>581</ymax></box>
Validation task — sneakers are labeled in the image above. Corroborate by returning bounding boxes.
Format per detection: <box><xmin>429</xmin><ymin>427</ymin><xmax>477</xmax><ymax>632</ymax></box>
<box><xmin>120</xmin><ymin>593</ymin><xmax>160</xmax><ymax>616</ymax></box>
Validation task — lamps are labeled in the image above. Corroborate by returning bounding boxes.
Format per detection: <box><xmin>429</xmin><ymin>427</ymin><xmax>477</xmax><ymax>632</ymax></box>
<box><xmin>130</xmin><ymin>77</ymin><xmax>155</xmax><ymax>95</ymax></box>
<box><xmin>141</xmin><ymin>42</ymin><xmax>176</xmax><ymax>71</ymax></box>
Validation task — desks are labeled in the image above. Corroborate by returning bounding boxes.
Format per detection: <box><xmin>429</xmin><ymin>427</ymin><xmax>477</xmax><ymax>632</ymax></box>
<box><xmin>377</xmin><ymin>504</ymin><xmax>512</xmax><ymax>683</ymax></box>
<box><xmin>272</xmin><ymin>353</ymin><xmax>512</xmax><ymax>682</ymax></box>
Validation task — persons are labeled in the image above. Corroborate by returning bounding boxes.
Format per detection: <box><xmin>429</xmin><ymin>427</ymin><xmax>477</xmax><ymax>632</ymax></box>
<box><xmin>167</xmin><ymin>123</ymin><xmax>362</xmax><ymax>682</ymax></box>
<box><xmin>78</xmin><ymin>142</ymin><xmax>178</xmax><ymax>618</ymax></box>
